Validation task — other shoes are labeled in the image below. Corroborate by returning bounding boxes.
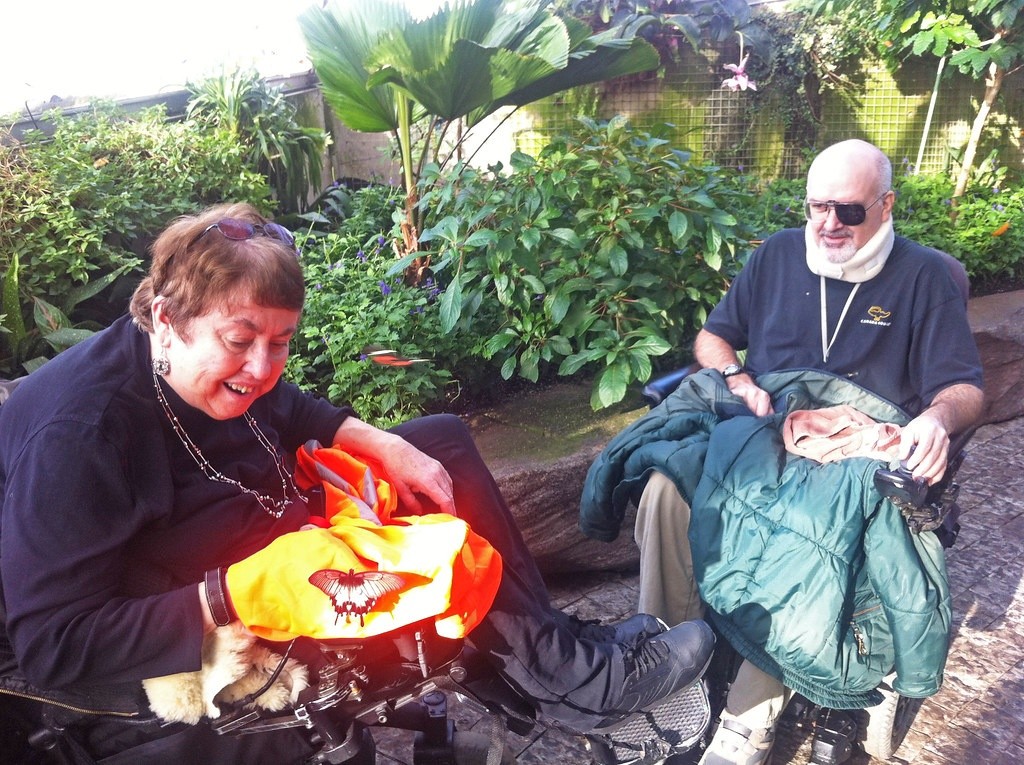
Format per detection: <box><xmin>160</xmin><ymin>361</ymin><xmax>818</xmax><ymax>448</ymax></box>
<box><xmin>700</xmin><ymin>713</ymin><xmax>777</xmax><ymax>765</ymax></box>
<box><xmin>588</xmin><ymin>618</ymin><xmax>716</xmax><ymax>735</ymax></box>
<box><xmin>567</xmin><ymin>613</ymin><xmax>665</xmax><ymax>645</ymax></box>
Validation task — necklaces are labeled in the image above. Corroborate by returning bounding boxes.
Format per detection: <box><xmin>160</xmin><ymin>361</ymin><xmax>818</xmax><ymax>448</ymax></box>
<box><xmin>816</xmin><ymin>274</ymin><xmax>861</xmax><ymax>363</ymax></box>
<box><xmin>146</xmin><ymin>356</ymin><xmax>309</xmax><ymax>519</ymax></box>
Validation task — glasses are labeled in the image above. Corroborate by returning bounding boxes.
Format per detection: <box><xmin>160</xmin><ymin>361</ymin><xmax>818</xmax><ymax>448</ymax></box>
<box><xmin>185</xmin><ymin>218</ymin><xmax>297</xmax><ymax>254</ymax></box>
<box><xmin>802</xmin><ymin>191</ymin><xmax>889</xmax><ymax>226</ymax></box>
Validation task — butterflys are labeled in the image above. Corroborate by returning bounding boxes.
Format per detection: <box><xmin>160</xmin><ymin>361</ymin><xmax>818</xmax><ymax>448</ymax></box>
<box><xmin>308</xmin><ymin>564</ymin><xmax>402</xmax><ymax>627</ymax></box>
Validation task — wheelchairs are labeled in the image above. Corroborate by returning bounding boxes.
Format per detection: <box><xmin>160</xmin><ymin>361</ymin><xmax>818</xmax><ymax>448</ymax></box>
<box><xmin>642</xmin><ymin>362</ymin><xmax>977</xmax><ymax>765</ymax></box>
<box><xmin>0</xmin><ymin>612</ymin><xmax>710</xmax><ymax>765</ymax></box>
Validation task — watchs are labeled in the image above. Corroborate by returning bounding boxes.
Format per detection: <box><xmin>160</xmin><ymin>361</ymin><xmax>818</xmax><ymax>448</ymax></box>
<box><xmin>722</xmin><ymin>364</ymin><xmax>746</xmax><ymax>380</ymax></box>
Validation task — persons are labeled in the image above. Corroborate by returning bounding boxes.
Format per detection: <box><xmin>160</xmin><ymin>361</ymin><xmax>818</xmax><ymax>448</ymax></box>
<box><xmin>0</xmin><ymin>200</ymin><xmax>716</xmax><ymax>736</ymax></box>
<box><xmin>634</xmin><ymin>137</ymin><xmax>986</xmax><ymax>712</ymax></box>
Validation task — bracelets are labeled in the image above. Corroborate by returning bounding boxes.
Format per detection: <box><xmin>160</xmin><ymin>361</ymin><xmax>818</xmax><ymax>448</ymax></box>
<box><xmin>204</xmin><ymin>563</ymin><xmax>237</xmax><ymax>629</ymax></box>
